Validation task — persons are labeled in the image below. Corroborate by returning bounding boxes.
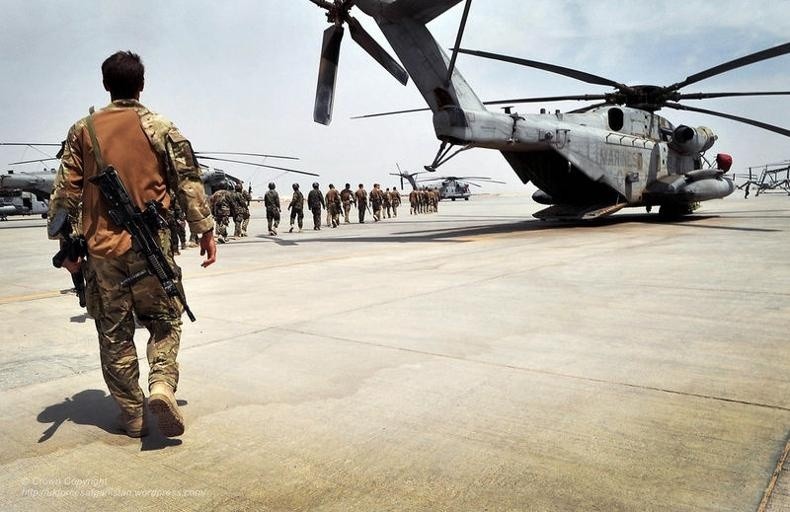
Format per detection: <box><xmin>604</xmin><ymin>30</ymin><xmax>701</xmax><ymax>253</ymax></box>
<box><xmin>47</xmin><ymin>51</ymin><xmax>216</xmax><ymax>438</ymax></box>
<box><xmin>164</xmin><ymin>182</ymin><xmax>440</xmax><ymax>256</ymax></box>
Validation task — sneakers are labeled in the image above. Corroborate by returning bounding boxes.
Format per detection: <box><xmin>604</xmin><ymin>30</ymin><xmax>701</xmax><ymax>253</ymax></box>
<box><xmin>314</xmin><ymin>225</ymin><xmax>321</xmax><ymax>230</ymax></box>
<box><xmin>218</xmin><ymin>233</ymin><xmax>247</xmax><ymax>243</ymax></box>
<box><xmin>289</xmin><ymin>228</ymin><xmax>294</xmax><ymax>232</ymax></box>
<box><xmin>373</xmin><ymin>215</ymin><xmax>380</xmax><ymax>221</ymax></box>
<box><xmin>328</xmin><ymin>219</ymin><xmax>350</xmax><ymax>227</ymax></box>
<box><xmin>117</xmin><ymin>414</ymin><xmax>149</xmax><ymax>437</ymax></box>
<box><xmin>270</xmin><ymin>227</ymin><xmax>277</xmax><ymax>235</ymax></box>
<box><xmin>149</xmin><ymin>382</ymin><xmax>184</xmax><ymax>437</ymax></box>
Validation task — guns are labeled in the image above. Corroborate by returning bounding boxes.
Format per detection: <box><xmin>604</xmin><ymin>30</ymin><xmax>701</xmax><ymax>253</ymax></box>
<box><xmin>334</xmin><ymin>200</ymin><xmax>345</xmax><ymax>217</ymax></box>
<box><xmin>50</xmin><ymin>211</ymin><xmax>86</xmax><ymax>309</ymax></box>
<box><xmin>247</xmin><ymin>182</ymin><xmax>251</xmax><ymax>206</ymax></box>
<box><xmin>350</xmin><ymin>199</ymin><xmax>357</xmax><ymax>209</ymax></box>
<box><xmin>363</xmin><ymin>201</ymin><xmax>371</xmax><ymax>215</ymax></box>
<box><xmin>89</xmin><ymin>164</ymin><xmax>197</xmax><ymax>322</ymax></box>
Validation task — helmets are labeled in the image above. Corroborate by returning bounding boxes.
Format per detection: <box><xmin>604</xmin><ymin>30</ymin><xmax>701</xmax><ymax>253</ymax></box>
<box><xmin>313</xmin><ymin>183</ymin><xmax>318</xmax><ymax>188</ymax></box>
<box><xmin>219</xmin><ymin>181</ymin><xmax>243</xmax><ymax>190</ymax></box>
<box><xmin>269</xmin><ymin>183</ymin><xmax>275</xmax><ymax>189</ymax></box>
<box><xmin>293</xmin><ymin>184</ymin><xmax>299</xmax><ymax>190</ymax></box>
